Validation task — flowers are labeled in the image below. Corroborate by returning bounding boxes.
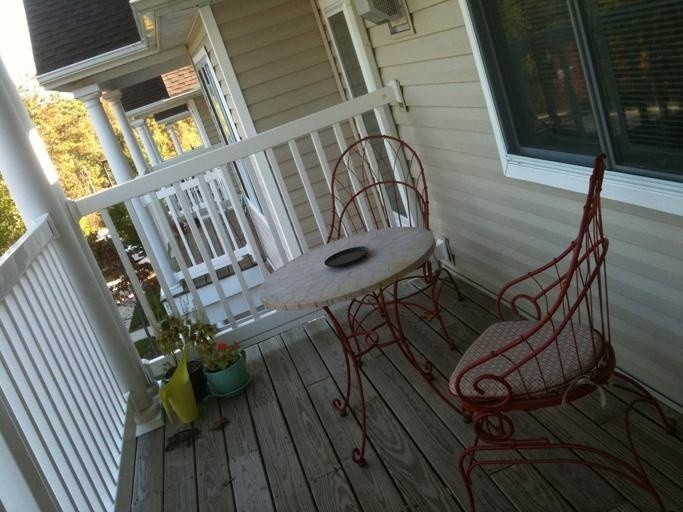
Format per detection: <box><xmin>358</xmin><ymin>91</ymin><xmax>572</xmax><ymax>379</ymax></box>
<box><xmin>191</xmin><ymin>322</ymin><xmax>242</xmax><ymax>370</ymax></box>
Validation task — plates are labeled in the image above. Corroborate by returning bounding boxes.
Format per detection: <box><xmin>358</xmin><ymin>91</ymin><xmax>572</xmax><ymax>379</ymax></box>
<box><xmin>324</xmin><ymin>245</ymin><xmax>369</xmax><ymax>268</ymax></box>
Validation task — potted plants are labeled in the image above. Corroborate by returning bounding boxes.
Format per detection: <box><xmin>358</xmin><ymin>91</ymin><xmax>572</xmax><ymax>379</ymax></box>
<box><xmin>146</xmin><ymin>294</ymin><xmax>207</xmax><ymax>405</ymax></box>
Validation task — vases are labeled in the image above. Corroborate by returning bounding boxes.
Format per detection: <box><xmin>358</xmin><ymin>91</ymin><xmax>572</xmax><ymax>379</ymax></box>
<box><xmin>201</xmin><ymin>350</ymin><xmax>252</xmax><ymax>398</ymax></box>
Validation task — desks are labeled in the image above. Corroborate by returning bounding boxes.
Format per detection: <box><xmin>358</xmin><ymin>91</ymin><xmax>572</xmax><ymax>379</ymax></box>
<box><xmin>257</xmin><ymin>224</ymin><xmax>474</xmax><ymax>467</ymax></box>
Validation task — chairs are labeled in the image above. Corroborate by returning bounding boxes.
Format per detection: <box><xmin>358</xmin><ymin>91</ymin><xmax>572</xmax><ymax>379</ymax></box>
<box><xmin>446</xmin><ymin>152</ymin><xmax>682</xmax><ymax>512</ymax></box>
<box><xmin>323</xmin><ymin>132</ymin><xmax>465</xmax><ymax>369</ymax></box>
